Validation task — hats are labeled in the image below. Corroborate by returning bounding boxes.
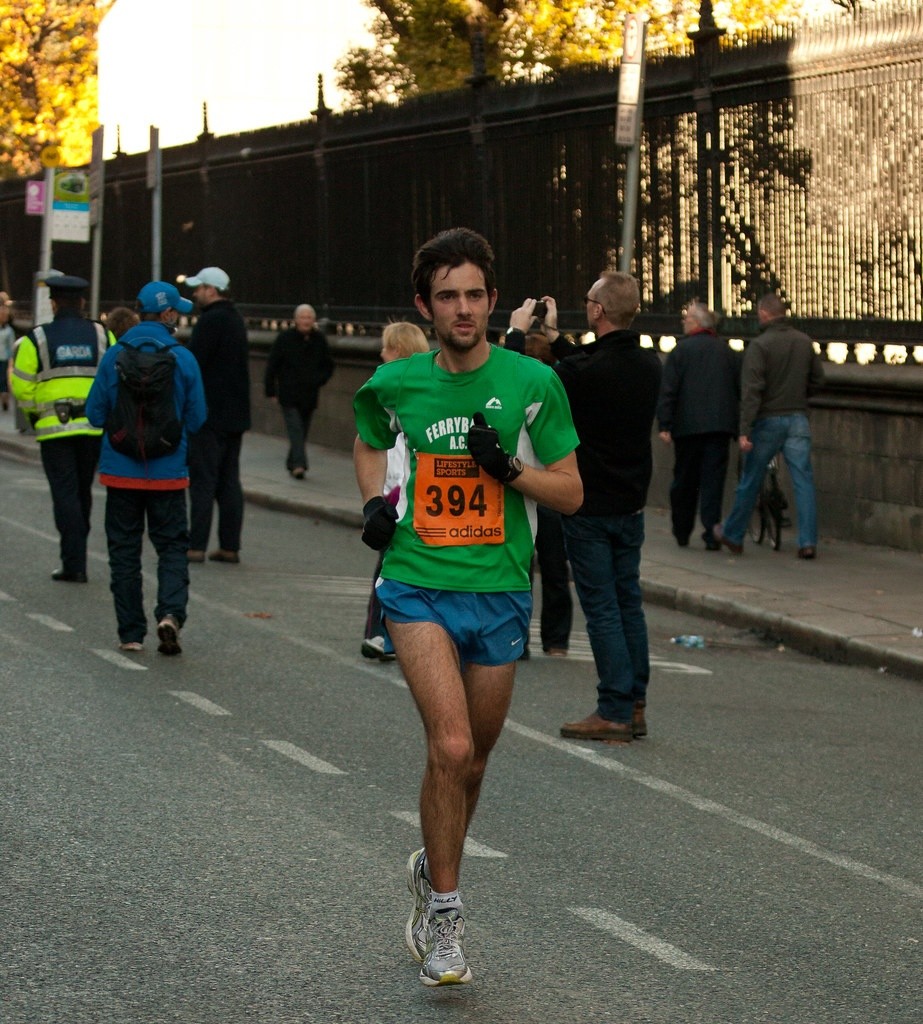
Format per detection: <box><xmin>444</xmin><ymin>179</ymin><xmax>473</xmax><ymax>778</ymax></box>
<box><xmin>185</xmin><ymin>267</ymin><xmax>230</xmax><ymax>292</ymax></box>
<box><xmin>136</xmin><ymin>281</ymin><xmax>193</xmax><ymax>314</ymax></box>
<box><xmin>45</xmin><ymin>276</ymin><xmax>90</xmax><ymax>299</ymax></box>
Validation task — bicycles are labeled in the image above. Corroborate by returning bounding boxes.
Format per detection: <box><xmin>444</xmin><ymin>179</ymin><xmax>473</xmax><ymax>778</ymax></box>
<box><xmin>747</xmin><ymin>453</ymin><xmax>793</xmax><ymax>551</ymax></box>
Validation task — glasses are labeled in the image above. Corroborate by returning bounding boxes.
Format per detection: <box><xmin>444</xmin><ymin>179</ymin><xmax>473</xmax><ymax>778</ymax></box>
<box><xmin>584</xmin><ymin>296</ymin><xmax>606</xmax><ymax>314</ymax></box>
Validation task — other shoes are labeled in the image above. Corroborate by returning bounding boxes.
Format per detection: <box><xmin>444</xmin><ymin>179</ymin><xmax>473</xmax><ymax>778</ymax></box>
<box><xmin>121</xmin><ymin>642</ymin><xmax>142</xmax><ymax>650</ymax></box>
<box><xmin>187</xmin><ymin>550</ymin><xmax>205</xmax><ymax>563</ymax></box>
<box><xmin>156</xmin><ymin>617</ymin><xmax>181</xmax><ymax>654</ymax></box>
<box><xmin>797</xmin><ymin>548</ymin><xmax>815</xmax><ymax>558</ymax></box>
<box><xmin>294</xmin><ymin>467</ymin><xmax>305</xmax><ymax>479</ymax></box>
<box><xmin>548</xmin><ymin>648</ymin><xmax>569</xmax><ymax>657</ymax></box>
<box><xmin>208</xmin><ymin>551</ymin><xmax>238</xmax><ymax>563</ymax></box>
<box><xmin>52</xmin><ymin>568</ymin><xmax>88</xmax><ymax>583</ymax></box>
<box><xmin>722</xmin><ymin>538</ymin><xmax>743</xmax><ymax>555</ymax></box>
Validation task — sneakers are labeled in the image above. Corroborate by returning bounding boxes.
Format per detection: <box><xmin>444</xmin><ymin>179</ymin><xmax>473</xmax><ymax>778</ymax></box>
<box><xmin>560</xmin><ymin>710</ymin><xmax>633</xmax><ymax>741</ymax></box>
<box><xmin>417</xmin><ymin>907</ymin><xmax>472</xmax><ymax>987</ymax></box>
<box><xmin>404</xmin><ymin>845</ymin><xmax>432</xmax><ymax>963</ymax></box>
<box><xmin>361</xmin><ymin>635</ymin><xmax>396</xmax><ymax>663</ymax></box>
<box><xmin>633</xmin><ymin>710</ymin><xmax>649</xmax><ymax>736</ymax></box>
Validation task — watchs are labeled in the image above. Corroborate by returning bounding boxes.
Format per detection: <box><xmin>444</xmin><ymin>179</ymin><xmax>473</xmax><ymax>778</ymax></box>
<box><xmin>504</xmin><ymin>455</ymin><xmax>523</xmax><ymax>486</ymax></box>
<box><xmin>506</xmin><ymin>327</ymin><xmax>526</xmax><ymax>335</ymax></box>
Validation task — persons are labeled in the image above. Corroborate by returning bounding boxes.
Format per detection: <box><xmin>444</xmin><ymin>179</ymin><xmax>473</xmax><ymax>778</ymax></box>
<box><xmin>263</xmin><ymin>304</ymin><xmax>336</xmax><ymax>478</ymax></box>
<box><xmin>362</xmin><ymin>318</ymin><xmax>429</xmax><ymax>662</ymax></box>
<box><xmin>504</xmin><ymin>270</ymin><xmax>664</xmax><ymax>742</ymax></box>
<box><xmin>0</xmin><ymin>290</ymin><xmax>16</xmax><ymax>413</ymax></box>
<box><xmin>713</xmin><ymin>295</ymin><xmax>826</xmax><ymax>560</ymax></box>
<box><xmin>355</xmin><ymin>227</ymin><xmax>584</xmax><ymax>987</ymax></box>
<box><xmin>86</xmin><ymin>282</ymin><xmax>209</xmax><ymax>656</ymax></box>
<box><xmin>184</xmin><ymin>267</ymin><xmax>252</xmax><ymax>564</ymax></box>
<box><xmin>7</xmin><ymin>275</ymin><xmax>119</xmax><ymax>582</ymax></box>
<box><xmin>98</xmin><ymin>303</ymin><xmax>140</xmax><ymax>343</ymax></box>
<box><xmin>658</xmin><ymin>302</ymin><xmax>745</xmax><ymax>553</ymax></box>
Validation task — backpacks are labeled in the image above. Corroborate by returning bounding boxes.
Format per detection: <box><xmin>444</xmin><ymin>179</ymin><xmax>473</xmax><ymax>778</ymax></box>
<box><xmin>104</xmin><ymin>342</ymin><xmax>189</xmax><ymax>463</ymax></box>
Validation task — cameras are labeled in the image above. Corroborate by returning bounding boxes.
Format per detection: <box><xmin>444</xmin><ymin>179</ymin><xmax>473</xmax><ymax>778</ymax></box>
<box><xmin>531</xmin><ymin>301</ymin><xmax>548</xmax><ymax>318</ymax></box>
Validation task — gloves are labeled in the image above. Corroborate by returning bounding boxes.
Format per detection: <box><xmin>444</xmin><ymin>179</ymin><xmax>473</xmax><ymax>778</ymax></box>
<box><xmin>468</xmin><ymin>412</ymin><xmax>510</xmax><ymax>481</ymax></box>
<box><xmin>361</xmin><ymin>496</ymin><xmax>398</xmax><ymax>550</ymax></box>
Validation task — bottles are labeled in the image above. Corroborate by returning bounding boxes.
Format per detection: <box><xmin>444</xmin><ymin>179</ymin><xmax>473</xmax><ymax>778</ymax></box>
<box><xmin>670</xmin><ymin>636</ymin><xmax>705</xmax><ymax>649</ymax></box>
